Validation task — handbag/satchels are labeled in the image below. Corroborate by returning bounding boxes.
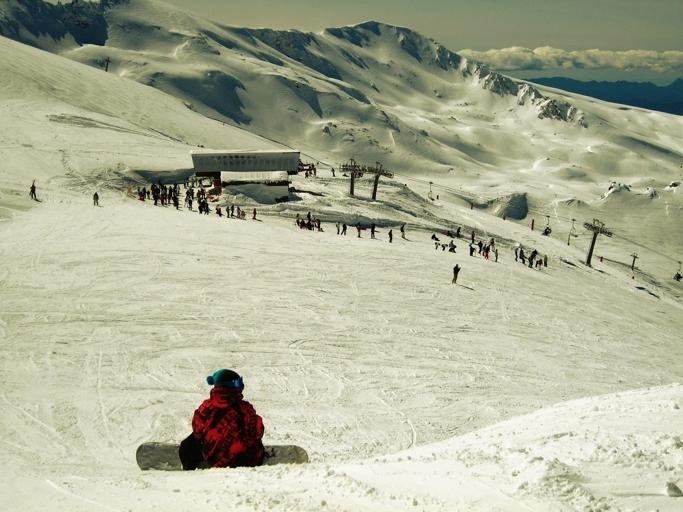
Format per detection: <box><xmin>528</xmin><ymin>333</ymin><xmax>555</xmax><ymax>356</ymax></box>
<box><xmin>178</xmin><ymin>432</ymin><xmax>203</xmax><ymax>470</ymax></box>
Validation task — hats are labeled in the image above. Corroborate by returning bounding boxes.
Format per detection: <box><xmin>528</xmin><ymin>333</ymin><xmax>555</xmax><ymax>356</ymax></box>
<box><xmin>207</xmin><ymin>369</ymin><xmax>244</xmax><ymax>389</ymax></box>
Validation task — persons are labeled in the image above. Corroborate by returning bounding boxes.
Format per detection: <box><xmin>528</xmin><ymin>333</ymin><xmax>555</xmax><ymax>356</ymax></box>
<box><xmin>387</xmin><ymin>229</ymin><xmax>392</xmax><ymax>243</ymax></box>
<box><xmin>451</xmin><ymin>263</ymin><xmax>461</xmax><ymax>284</ymax></box>
<box><xmin>428</xmin><ymin>226</ymin><xmax>549</xmax><ymax>272</ymax></box>
<box><xmin>135</xmin><ymin>174</ymin><xmax>257</xmax><ymax>221</ymax></box>
<box><xmin>303</xmin><ymin>163</ymin><xmax>315</xmax><ymax>179</ymax></box>
<box><xmin>177</xmin><ymin>367</ymin><xmax>265</xmax><ymax>471</ymax></box>
<box><xmin>399</xmin><ymin>224</ymin><xmax>405</xmax><ymax>239</ymax></box>
<box><xmin>29</xmin><ymin>184</ymin><xmax>36</xmax><ymax>199</ymax></box>
<box><xmin>92</xmin><ymin>191</ymin><xmax>99</xmax><ymax>206</ymax></box>
<box><xmin>293</xmin><ymin>210</ymin><xmax>376</xmax><ymax>239</ymax></box>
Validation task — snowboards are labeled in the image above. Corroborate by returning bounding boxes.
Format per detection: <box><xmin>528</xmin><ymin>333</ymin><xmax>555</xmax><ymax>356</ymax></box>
<box><xmin>137</xmin><ymin>441</ymin><xmax>308</xmax><ymax>470</ymax></box>
